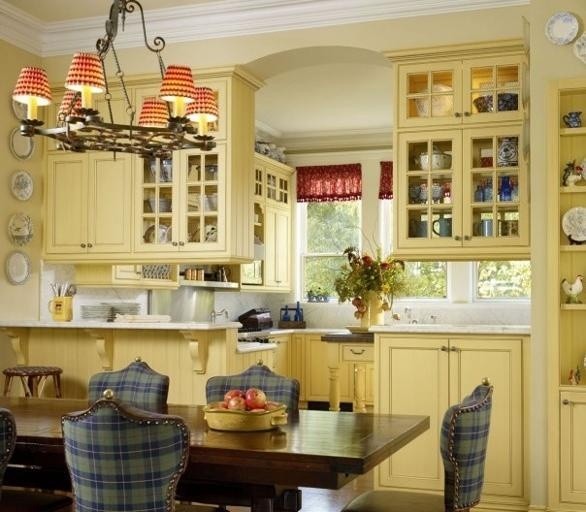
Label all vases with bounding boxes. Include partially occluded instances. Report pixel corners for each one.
[360,287,384,331]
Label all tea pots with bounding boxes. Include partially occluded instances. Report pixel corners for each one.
[216,264,231,282]
[562,112,583,127]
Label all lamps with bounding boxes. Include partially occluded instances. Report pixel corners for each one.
[11,1,217,159]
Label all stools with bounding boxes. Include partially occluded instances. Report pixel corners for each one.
[3,366,63,398]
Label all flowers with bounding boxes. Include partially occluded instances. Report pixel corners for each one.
[332,245,403,318]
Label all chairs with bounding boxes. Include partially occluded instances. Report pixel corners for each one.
[341,375,496,507]
[0,408,17,473]
[179,358,302,512]
[87,356,169,413]
[60,389,190,512]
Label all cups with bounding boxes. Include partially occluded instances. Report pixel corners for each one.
[191,268,198,281]
[499,220,517,236]
[198,267,204,281]
[185,267,191,281]
[479,219,499,236]
[433,217,452,236]
[409,218,427,237]
[256,140,288,164]
[46,296,72,322]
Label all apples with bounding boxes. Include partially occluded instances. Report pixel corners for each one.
[218,387,279,414]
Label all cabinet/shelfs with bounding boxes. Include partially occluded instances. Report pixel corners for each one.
[238,334,528,508]
[548,77,586,511]
[43,67,299,296]
[396,52,531,262]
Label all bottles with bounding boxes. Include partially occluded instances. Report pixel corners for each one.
[282,304,290,321]
[501,177,512,203]
[294,302,302,321]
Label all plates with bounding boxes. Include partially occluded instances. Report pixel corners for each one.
[572,30,586,66]
[7,127,36,161]
[5,249,31,288]
[9,98,27,121]
[144,224,168,242]
[6,212,32,247]
[166,224,216,241]
[79,301,140,322]
[114,312,172,324]
[9,170,33,202]
[543,12,579,45]
[414,83,456,117]
[561,206,586,243]
[499,141,519,167]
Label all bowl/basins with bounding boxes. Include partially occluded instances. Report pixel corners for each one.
[148,160,191,184]
[200,400,288,431]
[196,165,216,181]
[473,92,521,113]
[196,194,217,211]
[419,153,452,170]
[147,197,171,213]
